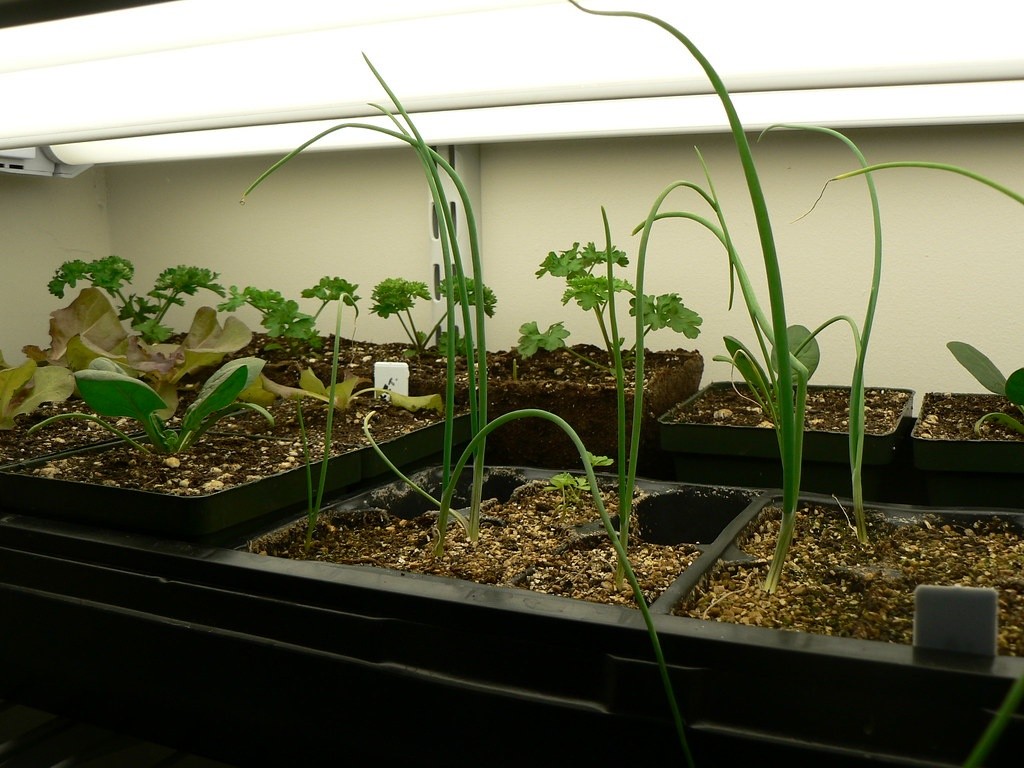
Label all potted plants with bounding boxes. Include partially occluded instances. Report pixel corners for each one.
[1,1,1024,768]
